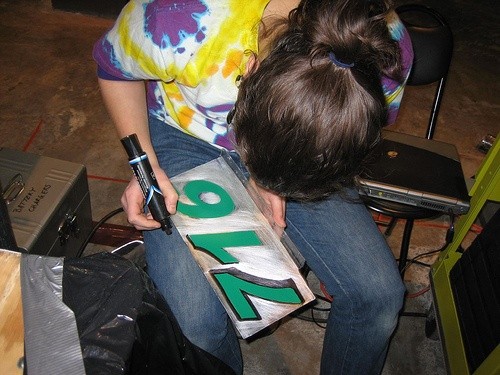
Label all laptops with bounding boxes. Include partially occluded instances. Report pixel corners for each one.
[356,127,470,215]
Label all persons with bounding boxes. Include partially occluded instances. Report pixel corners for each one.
[90,0,414,375]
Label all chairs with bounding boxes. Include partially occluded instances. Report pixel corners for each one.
[361,5,455,284]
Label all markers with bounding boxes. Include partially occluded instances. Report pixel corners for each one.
[120,132,174,236]
